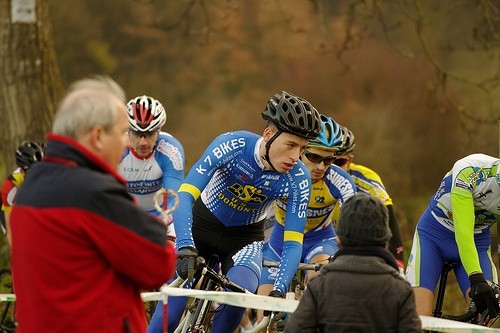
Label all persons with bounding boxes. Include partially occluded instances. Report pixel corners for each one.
[117,94,185,235]
[283,192,424,333]
[253,113,359,333]
[330,124,407,277]
[146,91,323,333]
[0,140,46,248]
[403,152,500,333]
[11,73,175,333]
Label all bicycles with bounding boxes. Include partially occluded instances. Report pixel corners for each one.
[159,254,287,333]
[261,256,335,302]
[0,267,17,333]
[427,258,500,329]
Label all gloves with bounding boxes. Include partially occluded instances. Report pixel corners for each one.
[177,246,199,283]
[468,272,500,318]
[264,290,285,324]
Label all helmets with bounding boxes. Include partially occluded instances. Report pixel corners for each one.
[332,125,355,157]
[308,113,343,150]
[15,141,46,173]
[261,90,322,140]
[126,95,166,132]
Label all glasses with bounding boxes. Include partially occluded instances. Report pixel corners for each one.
[303,150,336,165]
[131,129,159,137]
[333,156,351,167]
